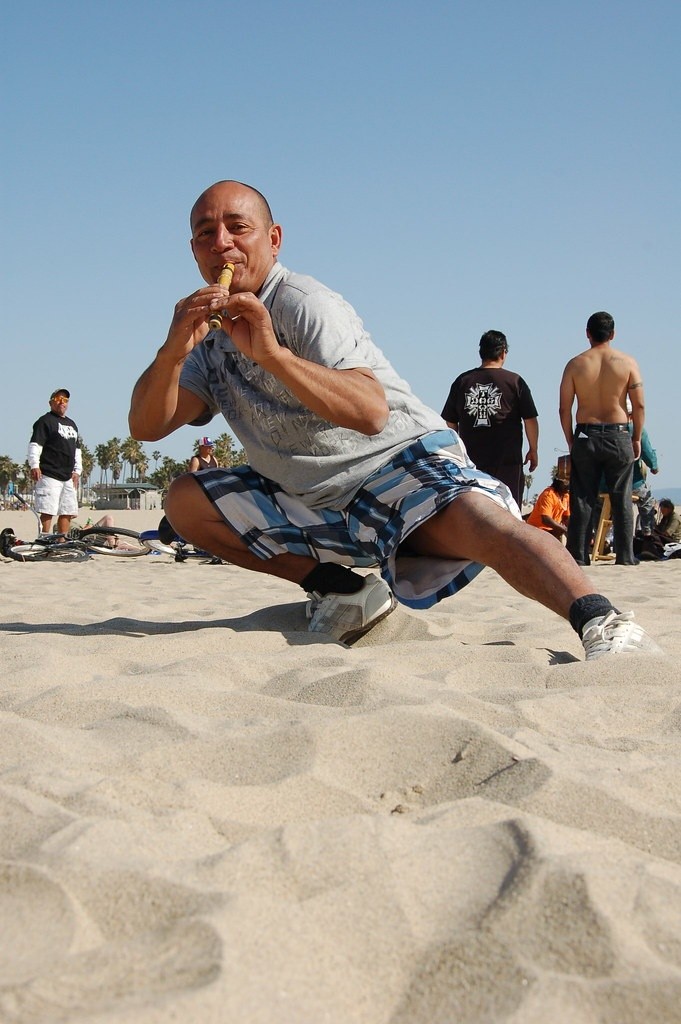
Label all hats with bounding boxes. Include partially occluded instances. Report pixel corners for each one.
[50,387,70,399]
[626,399,633,413]
[199,437,215,446]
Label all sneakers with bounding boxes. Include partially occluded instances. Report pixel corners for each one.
[305,573,398,645]
[581,609,649,661]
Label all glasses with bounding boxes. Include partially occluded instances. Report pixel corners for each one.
[51,396,69,403]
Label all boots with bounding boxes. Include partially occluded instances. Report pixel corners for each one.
[640,536,659,559]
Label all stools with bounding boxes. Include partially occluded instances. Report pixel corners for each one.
[593,494,639,562]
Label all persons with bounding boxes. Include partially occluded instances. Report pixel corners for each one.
[559,311,646,565]
[127,179,651,659]
[441,331,539,506]
[53,515,140,550]
[28,388,83,542]
[624,400,658,534]
[589,498,681,559]
[190,436,218,473]
[528,473,570,541]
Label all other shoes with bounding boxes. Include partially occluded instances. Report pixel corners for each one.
[575,559,589,566]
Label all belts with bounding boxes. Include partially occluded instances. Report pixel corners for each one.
[577,423,629,431]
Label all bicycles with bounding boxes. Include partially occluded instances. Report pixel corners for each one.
[0,492,152,562]
[138,530,224,564]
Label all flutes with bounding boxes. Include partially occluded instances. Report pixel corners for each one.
[208,262,235,331]
